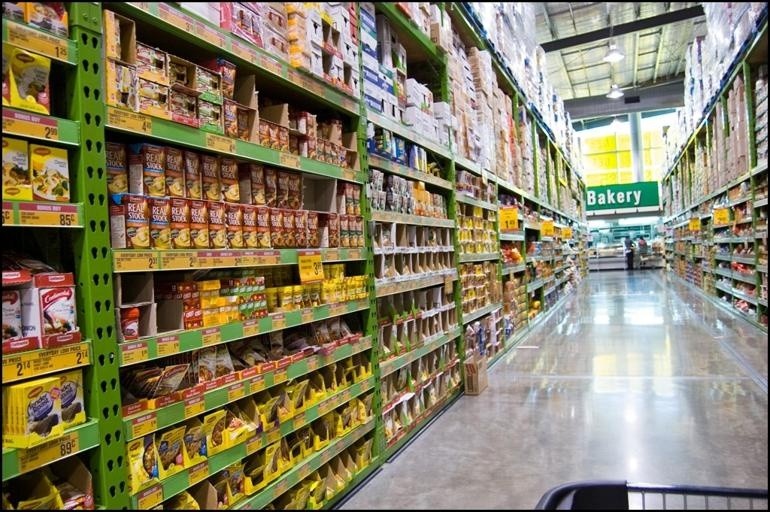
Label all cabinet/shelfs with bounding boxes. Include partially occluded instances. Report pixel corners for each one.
[656,1,768,329]
[1,1,588,511]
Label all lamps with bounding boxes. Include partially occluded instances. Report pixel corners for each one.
[602,0,625,64]
[607,65,626,100]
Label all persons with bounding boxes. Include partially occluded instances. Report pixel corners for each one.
[623,235,636,271]
[637,236,648,270]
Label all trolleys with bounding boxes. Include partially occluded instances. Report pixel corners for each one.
[537,480,769,510]
